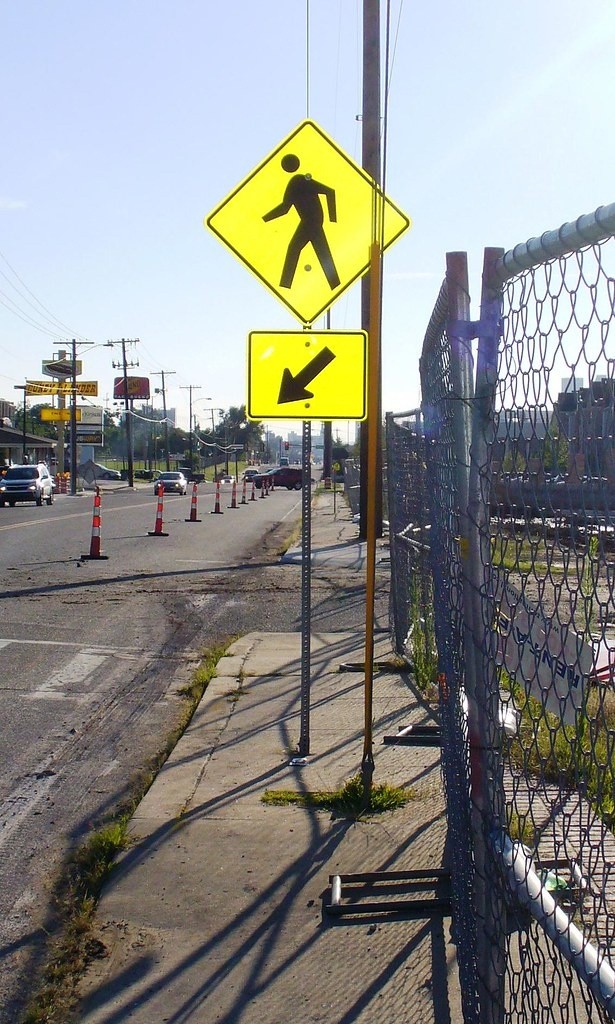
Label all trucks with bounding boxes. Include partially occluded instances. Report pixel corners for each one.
[178,467,205,483]
[280,456,290,466]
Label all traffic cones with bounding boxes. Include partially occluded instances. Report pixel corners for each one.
[134,469,162,480]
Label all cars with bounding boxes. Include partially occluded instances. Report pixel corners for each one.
[242,468,261,482]
[154,472,187,495]
[220,475,236,484]
[294,459,300,465]
[95,463,122,479]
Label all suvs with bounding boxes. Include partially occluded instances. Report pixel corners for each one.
[252,466,303,490]
[0,463,53,507]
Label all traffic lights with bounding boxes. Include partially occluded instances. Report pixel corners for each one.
[285,442,289,450]
